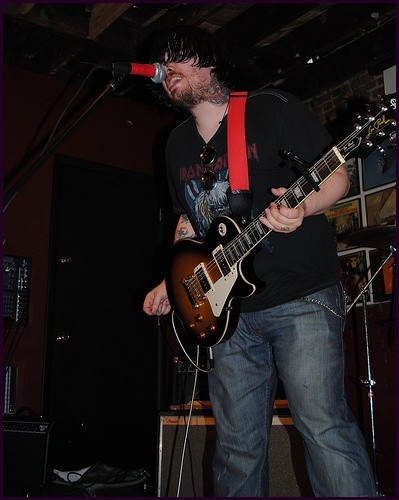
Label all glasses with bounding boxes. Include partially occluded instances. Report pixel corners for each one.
[199,145,218,190]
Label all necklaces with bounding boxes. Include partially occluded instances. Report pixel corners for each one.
[224,100,229,117]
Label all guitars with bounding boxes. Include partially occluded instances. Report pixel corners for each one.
[165,93,399,348]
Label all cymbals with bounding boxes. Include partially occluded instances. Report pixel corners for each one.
[339,225,399,249]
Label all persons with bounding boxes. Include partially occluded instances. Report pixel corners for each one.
[143,25,378,498]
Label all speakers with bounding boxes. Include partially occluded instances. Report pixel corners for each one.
[2,413,55,497]
[155,410,316,498]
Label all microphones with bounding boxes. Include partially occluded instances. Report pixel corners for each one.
[94,61,167,84]
